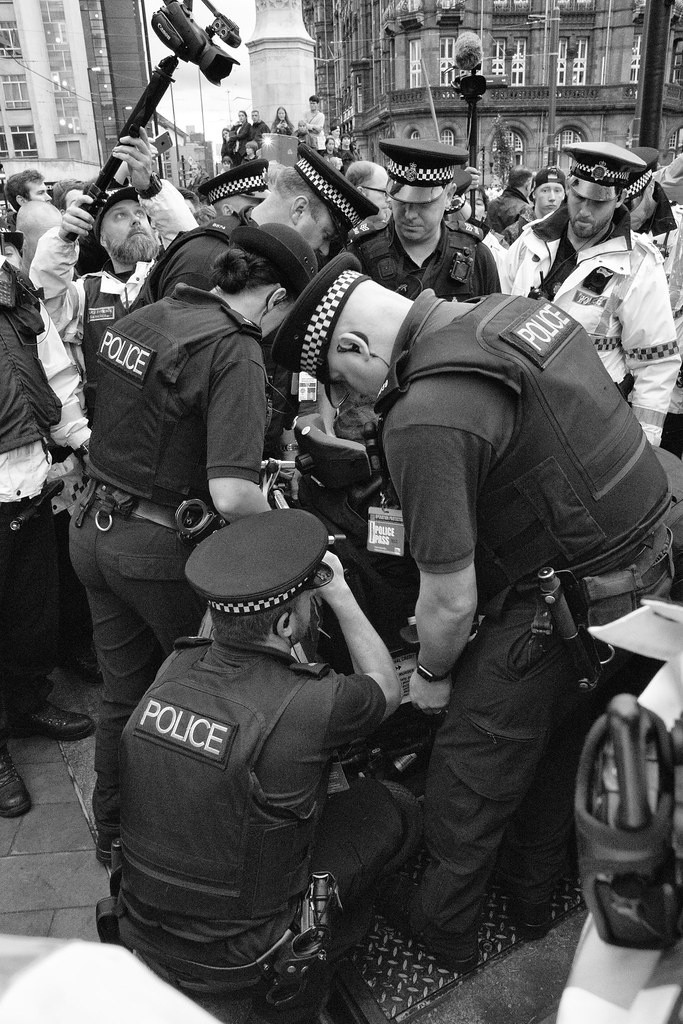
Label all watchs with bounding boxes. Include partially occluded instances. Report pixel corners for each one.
[135,172,162,198]
[416,657,452,682]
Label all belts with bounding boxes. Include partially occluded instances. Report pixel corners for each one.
[86,479,178,530]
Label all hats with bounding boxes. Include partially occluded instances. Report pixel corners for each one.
[378,139,469,203]
[620,147,658,201]
[197,159,270,203]
[443,169,471,222]
[230,224,319,286]
[529,168,566,201]
[94,185,139,240]
[270,252,370,409]
[329,126,339,135]
[293,144,380,252]
[562,142,647,202]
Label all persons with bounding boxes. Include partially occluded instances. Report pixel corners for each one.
[270,249,680,969]
[91,507,426,1024]
[1,94,682,866]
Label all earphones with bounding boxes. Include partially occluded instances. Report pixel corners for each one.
[283,618,289,629]
[347,345,360,354]
[272,295,289,307]
[616,189,623,202]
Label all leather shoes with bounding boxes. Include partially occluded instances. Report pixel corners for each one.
[0,739,29,816]
[501,857,547,939]
[378,872,481,973]
[5,700,93,741]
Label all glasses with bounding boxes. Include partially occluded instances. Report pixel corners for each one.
[358,186,388,197]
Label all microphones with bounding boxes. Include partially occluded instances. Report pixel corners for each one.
[453,31,484,71]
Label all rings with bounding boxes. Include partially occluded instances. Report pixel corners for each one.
[435,710,442,715]
